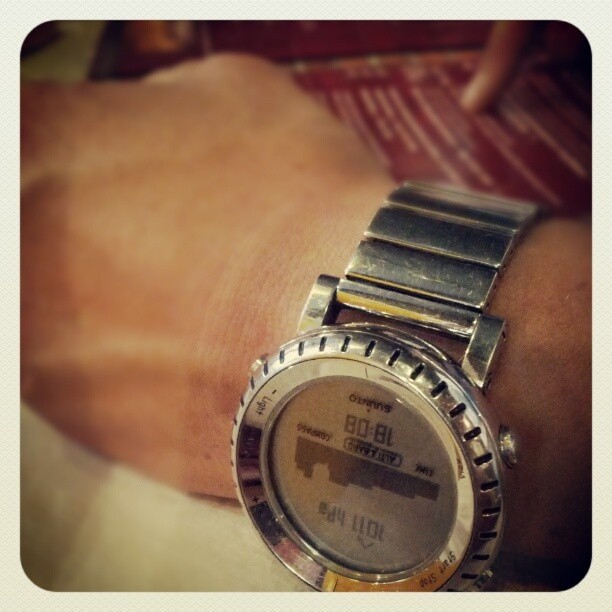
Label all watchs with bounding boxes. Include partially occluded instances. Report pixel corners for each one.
[231,174,551,592]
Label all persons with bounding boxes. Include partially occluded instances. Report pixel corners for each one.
[20,19,591,592]
